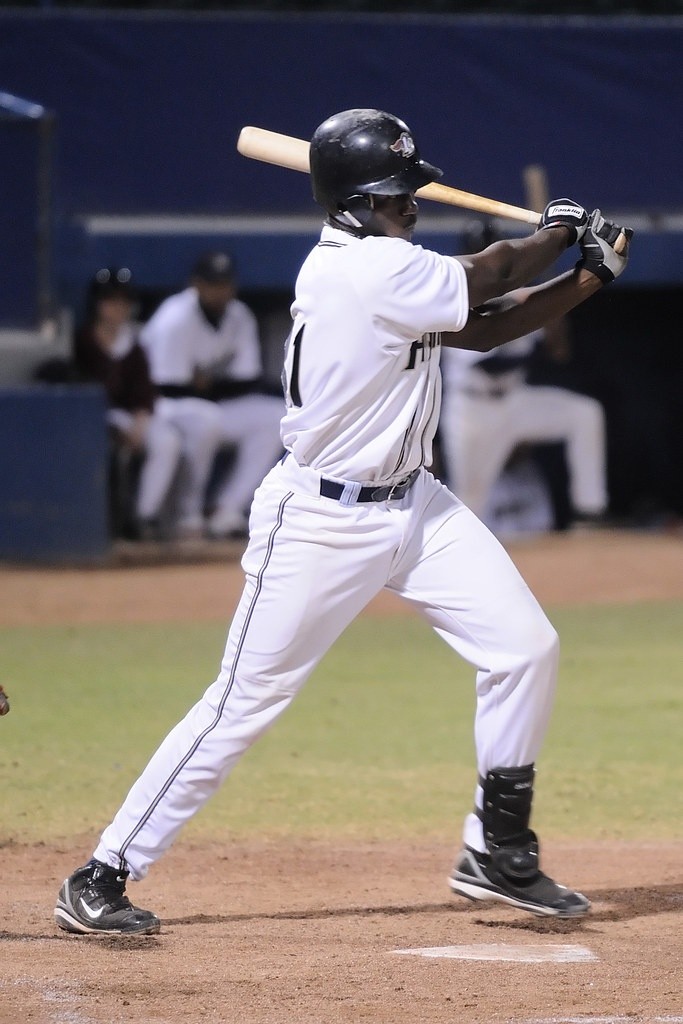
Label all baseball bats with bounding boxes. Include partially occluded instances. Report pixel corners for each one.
[236,126,627,255]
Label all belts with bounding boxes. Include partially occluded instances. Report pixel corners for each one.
[281,451,421,502]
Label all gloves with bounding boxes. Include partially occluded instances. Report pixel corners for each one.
[535,197,590,246]
[577,209,635,286]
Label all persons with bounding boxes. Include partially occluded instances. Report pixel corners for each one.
[68,263,179,543]
[53,109,636,934]
[139,245,294,536]
[440,219,613,529]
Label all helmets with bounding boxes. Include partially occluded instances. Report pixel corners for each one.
[197,252,238,281]
[309,109,445,213]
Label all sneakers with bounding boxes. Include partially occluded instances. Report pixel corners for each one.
[449,844,592,916]
[53,864,161,933]
